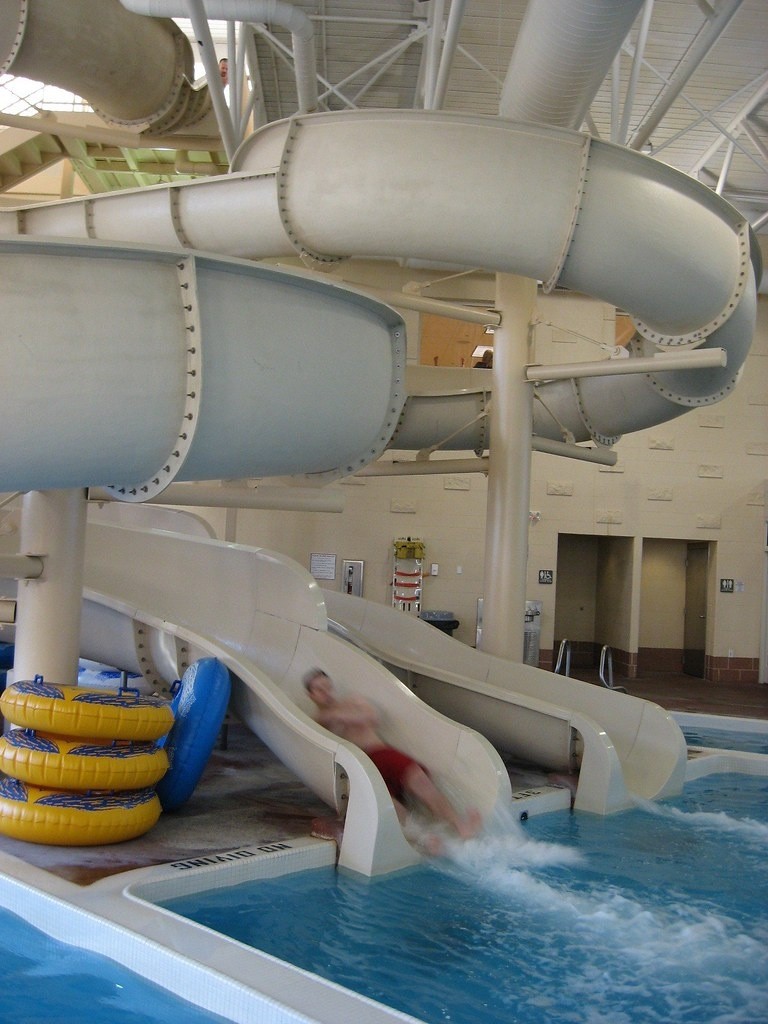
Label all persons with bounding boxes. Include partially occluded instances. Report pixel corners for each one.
[473,350,493,368]
[219,57,251,92]
[303,668,483,860]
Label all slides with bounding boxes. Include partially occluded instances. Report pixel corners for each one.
[0,1,757,880]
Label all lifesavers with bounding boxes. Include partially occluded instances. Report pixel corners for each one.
[0,774,162,845]
[0,727,167,790]
[165,656,232,811]
[1,676,172,739]
